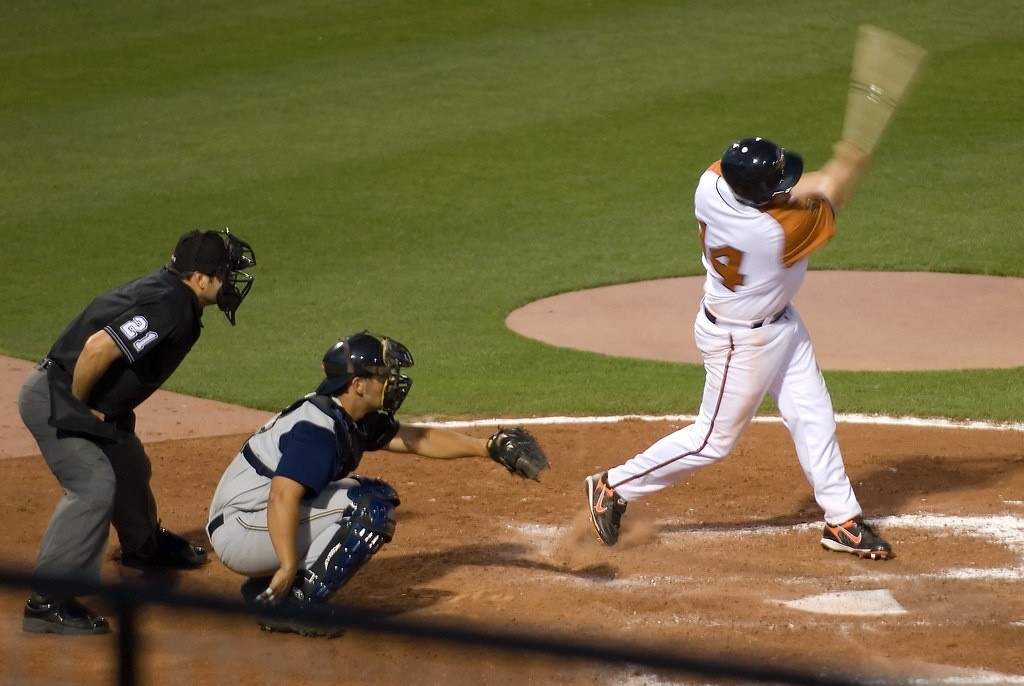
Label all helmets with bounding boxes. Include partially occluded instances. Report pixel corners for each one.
[720,139,804,198]
[315,334,394,394]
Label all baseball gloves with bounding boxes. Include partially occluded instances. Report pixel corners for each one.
[487,428,547,481]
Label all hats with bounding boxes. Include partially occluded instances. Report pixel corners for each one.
[170,229,228,274]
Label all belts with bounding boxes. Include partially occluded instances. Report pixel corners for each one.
[704,302,789,328]
[208,512,224,539]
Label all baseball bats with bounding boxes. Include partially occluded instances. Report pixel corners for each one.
[839,22,927,154]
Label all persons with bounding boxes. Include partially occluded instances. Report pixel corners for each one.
[205,331,521,641]
[585,139,894,560]
[14,226,257,633]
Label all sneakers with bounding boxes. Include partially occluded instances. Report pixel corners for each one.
[259,599,340,637]
[821,516,892,561]
[583,470,627,546]
[24,594,111,637]
[119,526,208,570]
